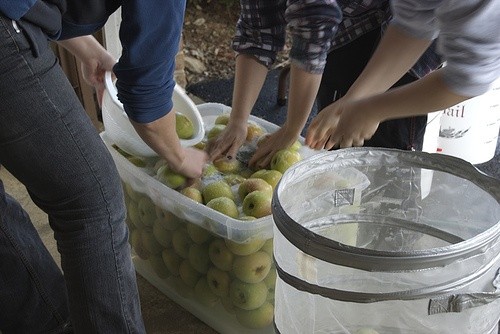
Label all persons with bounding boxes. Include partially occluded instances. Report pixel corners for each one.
[216,0,426,165]
[0,0,212,333]
[305,0,500,150]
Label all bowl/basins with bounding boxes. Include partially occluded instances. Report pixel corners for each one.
[101,69,205,159]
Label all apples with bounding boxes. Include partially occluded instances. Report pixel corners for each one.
[110,112,301,330]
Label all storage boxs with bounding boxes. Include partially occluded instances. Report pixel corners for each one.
[97,101,372,333]
